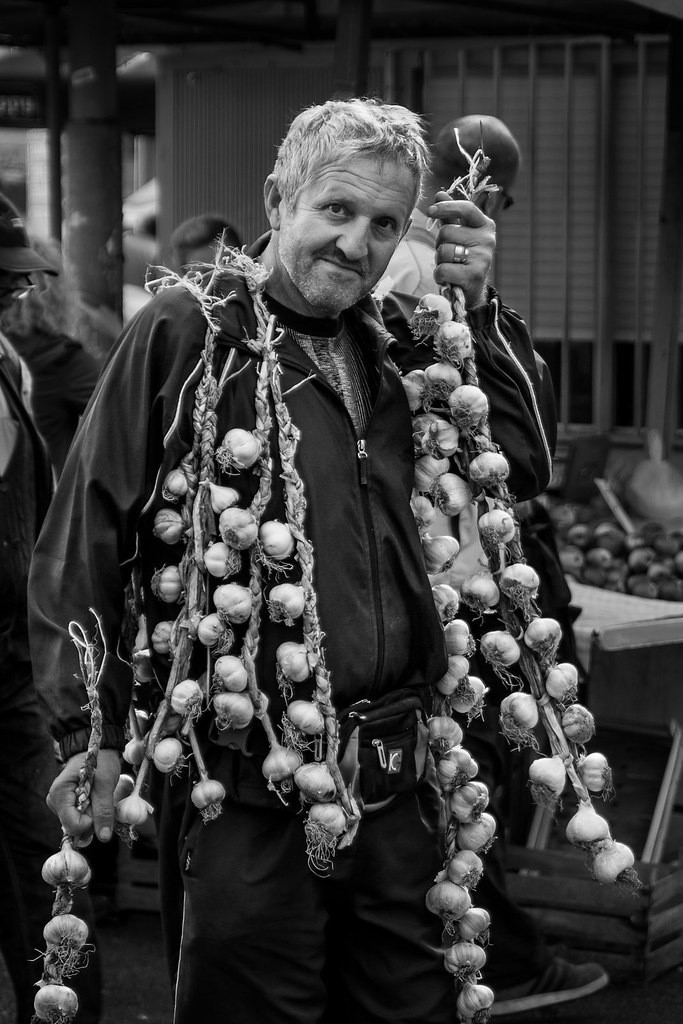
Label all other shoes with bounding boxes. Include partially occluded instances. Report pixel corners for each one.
[486,956,609,1017]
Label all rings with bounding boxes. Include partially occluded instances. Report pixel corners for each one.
[454,244,468,263]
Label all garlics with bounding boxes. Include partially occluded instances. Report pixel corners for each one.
[396,293,641,1024]
[34,426,347,1024]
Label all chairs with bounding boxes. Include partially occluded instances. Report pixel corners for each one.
[544,436,608,503]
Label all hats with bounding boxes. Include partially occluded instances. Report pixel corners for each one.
[0,192,59,278]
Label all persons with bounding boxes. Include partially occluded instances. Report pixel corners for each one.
[0,196,105,1024]
[27,98,609,1024]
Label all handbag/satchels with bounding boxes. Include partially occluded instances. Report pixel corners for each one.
[332,687,432,812]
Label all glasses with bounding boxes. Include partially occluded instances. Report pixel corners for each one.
[0,276,37,300]
[499,190,514,210]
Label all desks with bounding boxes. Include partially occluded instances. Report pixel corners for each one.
[510,575,683,879]
